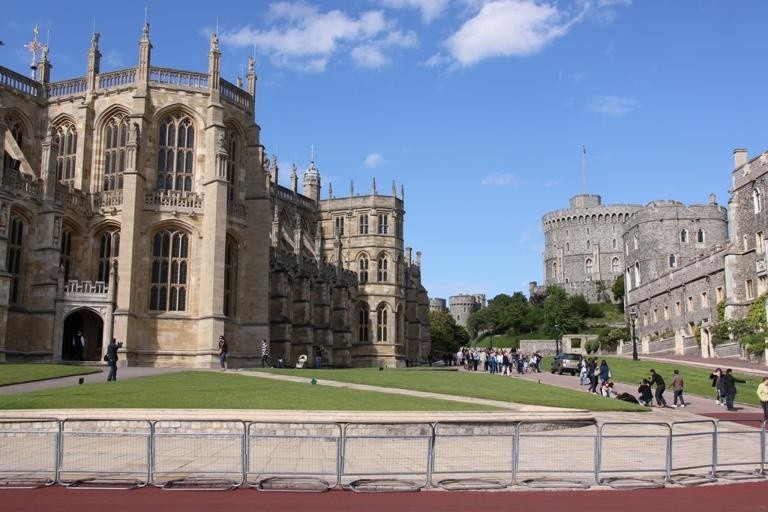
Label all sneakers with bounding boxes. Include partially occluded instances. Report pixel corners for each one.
[670,405,676,408]
[680,405,684,408]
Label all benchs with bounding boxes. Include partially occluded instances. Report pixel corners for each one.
[284,360,291,368]
[316,362,345,369]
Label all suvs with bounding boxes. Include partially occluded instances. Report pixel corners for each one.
[550,353,583,376]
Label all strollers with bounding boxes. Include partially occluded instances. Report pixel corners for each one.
[295,354,307,369]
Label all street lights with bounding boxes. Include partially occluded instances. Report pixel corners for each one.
[554,323,559,355]
[489,328,493,348]
[629,309,638,361]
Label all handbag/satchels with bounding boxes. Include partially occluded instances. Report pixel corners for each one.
[104,355,108,361]
[593,368,600,376]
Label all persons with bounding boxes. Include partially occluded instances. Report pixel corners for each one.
[576,355,639,405]
[708,368,726,407]
[216,335,227,372]
[442,352,447,366]
[260,338,274,371]
[297,355,305,364]
[715,373,728,407]
[452,345,544,377]
[667,369,684,408]
[105,337,123,383]
[276,356,284,369]
[427,351,432,367]
[637,378,653,406]
[315,346,322,369]
[647,368,667,408]
[722,368,748,411]
[71,329,85,360]
[755,376,768,425]
[447,351,452,367]
